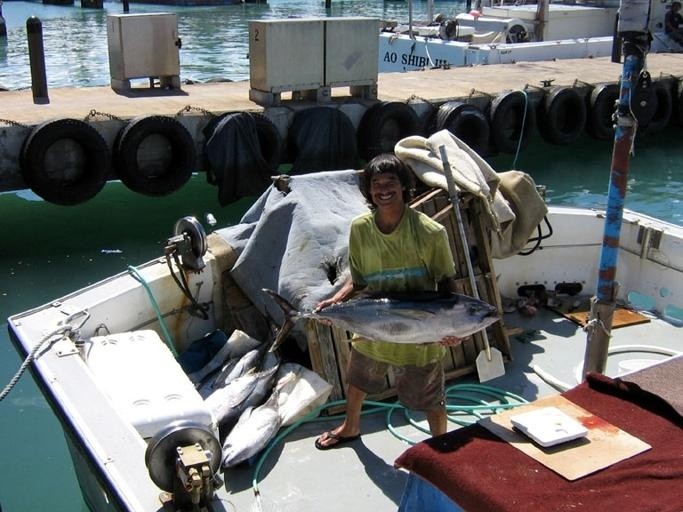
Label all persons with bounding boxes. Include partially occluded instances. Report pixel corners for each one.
[306,151,472,453]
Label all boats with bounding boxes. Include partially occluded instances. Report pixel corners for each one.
[7,207,683,512]
[378,0,683,74]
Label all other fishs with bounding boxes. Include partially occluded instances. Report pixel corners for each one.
[260,288,503,353]
[188,305,303,469]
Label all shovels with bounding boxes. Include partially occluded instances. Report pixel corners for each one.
[439,145,506,384]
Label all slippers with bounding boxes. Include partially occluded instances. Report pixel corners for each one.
[315,430,361,450]
